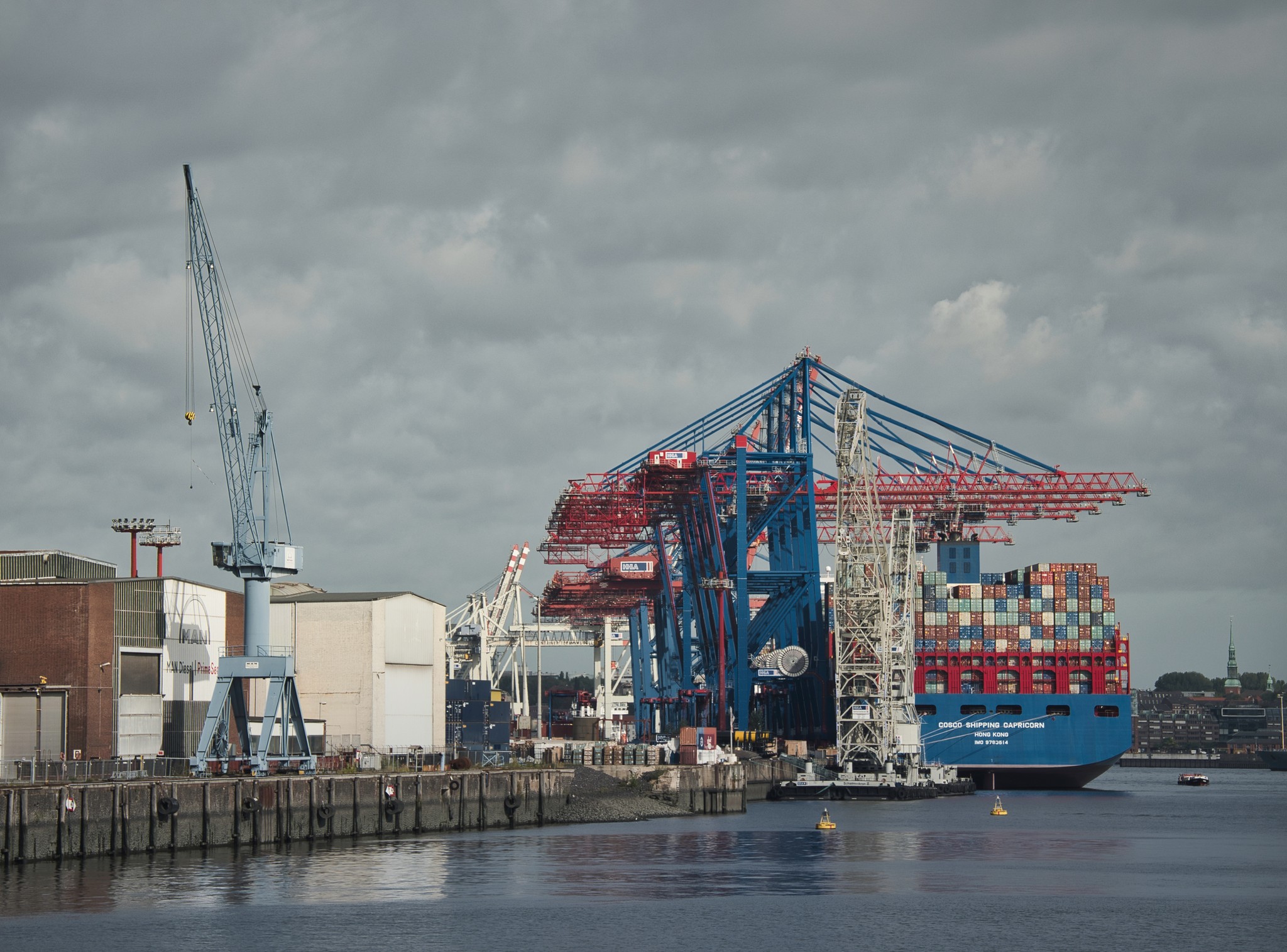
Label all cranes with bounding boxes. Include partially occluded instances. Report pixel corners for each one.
[444,348,1155,802]
[171,164,316,778]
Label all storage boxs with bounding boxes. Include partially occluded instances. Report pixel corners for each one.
[14,679,716,786]
[820,563,1116,694]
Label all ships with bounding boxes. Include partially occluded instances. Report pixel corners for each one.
[823,559,1133,791]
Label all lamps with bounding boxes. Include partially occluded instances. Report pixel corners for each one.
[99,662,110,668]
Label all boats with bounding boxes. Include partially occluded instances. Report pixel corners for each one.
[1178,774,1210,787]
